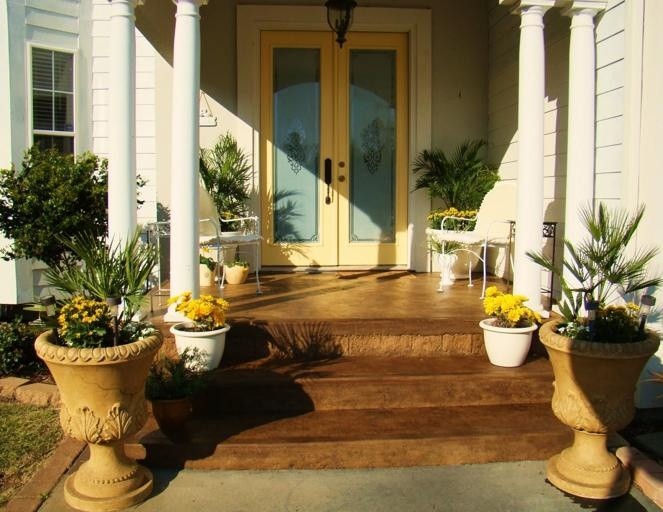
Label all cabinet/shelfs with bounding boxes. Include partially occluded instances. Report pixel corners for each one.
[504,220,558,309]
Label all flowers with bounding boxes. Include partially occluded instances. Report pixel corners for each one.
[24,223,159,349]
[521,198,663,343]
[158,290,230,331]
[481,286,542,327]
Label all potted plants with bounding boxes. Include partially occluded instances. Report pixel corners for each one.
[145,347,209,432]
[406,137,500,285]
[198,130,251,286]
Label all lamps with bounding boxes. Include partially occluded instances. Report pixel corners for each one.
[324,0,359,49]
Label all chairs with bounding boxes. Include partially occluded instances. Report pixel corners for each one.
[436,181,518,300]
[199,173,264,300]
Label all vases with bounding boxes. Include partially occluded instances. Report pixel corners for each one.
[479,318,538,368]
[539,316,660,499]
[169,322,230,373]
[35,319,164,512]
